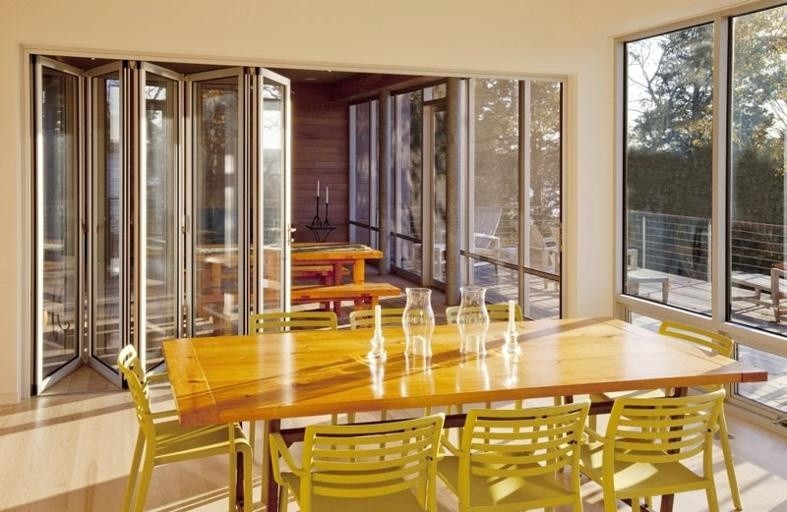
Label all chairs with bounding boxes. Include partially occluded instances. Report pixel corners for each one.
[732,264,787,323]
[412,204,560,305]
[625,248,671,302]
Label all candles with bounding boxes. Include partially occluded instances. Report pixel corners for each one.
[314,176,330,205]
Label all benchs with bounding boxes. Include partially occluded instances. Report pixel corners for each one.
[194,240,402,335]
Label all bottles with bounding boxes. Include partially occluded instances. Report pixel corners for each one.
[402,287,435,360]
[368,305,387,363]
[503,300,521,355]
[456,285,491,357]
